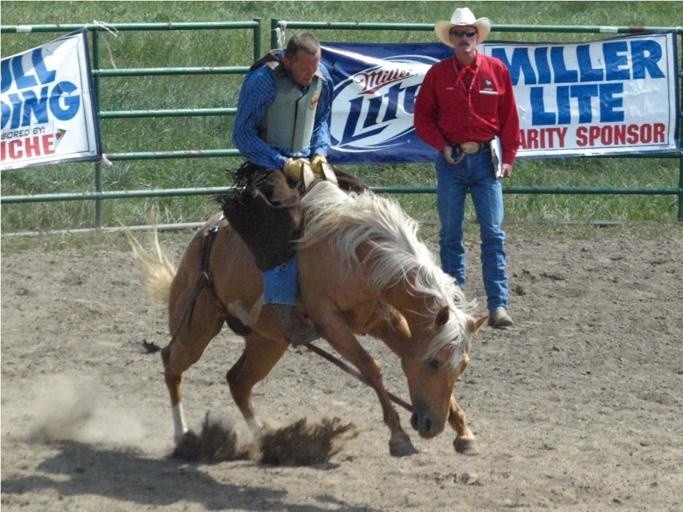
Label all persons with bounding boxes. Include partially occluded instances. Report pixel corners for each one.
[411,6,520,330]
[229,30,372,344]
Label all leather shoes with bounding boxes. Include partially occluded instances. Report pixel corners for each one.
[489,306,512,325]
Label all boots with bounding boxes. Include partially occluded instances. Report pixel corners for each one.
[273,305,319,344]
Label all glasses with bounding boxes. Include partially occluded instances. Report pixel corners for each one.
[452,30,475,37]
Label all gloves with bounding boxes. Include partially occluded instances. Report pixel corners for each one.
[283,157,311,181]
[311,156,327,173]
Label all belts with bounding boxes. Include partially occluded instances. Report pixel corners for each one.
[453,142,489,153]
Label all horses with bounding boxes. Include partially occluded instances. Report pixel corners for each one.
[111,177,492,457]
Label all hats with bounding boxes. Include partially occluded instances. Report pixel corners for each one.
[435,7,491,47]
